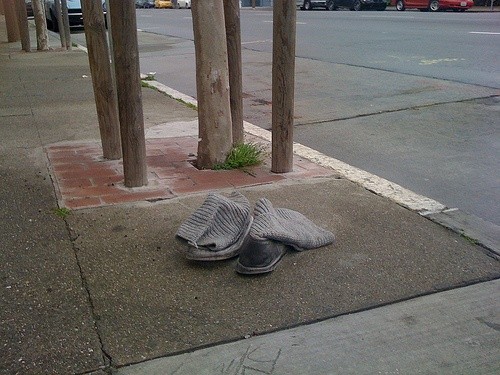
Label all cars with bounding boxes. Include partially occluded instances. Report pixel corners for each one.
[46,0,110,33]
[390,0,475,12]
[296,0,327,10]
[136,0,191,9]
[325,0,387,12]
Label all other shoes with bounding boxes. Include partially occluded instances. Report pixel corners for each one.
[174,189,336,276]
